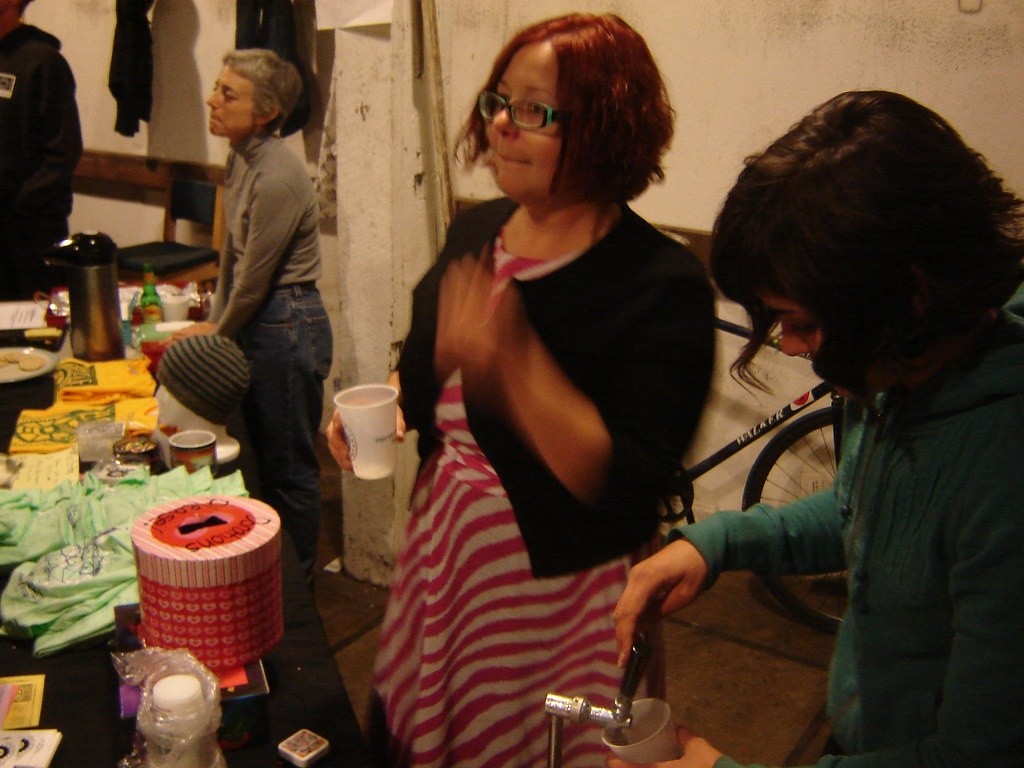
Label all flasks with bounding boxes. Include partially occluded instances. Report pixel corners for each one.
[65,231,126,363]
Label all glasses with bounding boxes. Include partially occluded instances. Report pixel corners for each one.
[478,92,564,129]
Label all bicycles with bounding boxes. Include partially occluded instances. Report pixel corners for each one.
[651,307,850,635]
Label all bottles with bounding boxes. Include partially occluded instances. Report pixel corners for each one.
[141,263,163,323]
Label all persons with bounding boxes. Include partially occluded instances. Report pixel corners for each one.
[155,336,247,465]
[325,14,715,768]
[0,0,84,302]
[159,48,334,628]
[605,90,1024,768]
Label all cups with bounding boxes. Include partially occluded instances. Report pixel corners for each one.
[334,385,399,479]
[150,675,220,768]
[601,697,678,764]
[114,434,160,476]
[169,432,217,475]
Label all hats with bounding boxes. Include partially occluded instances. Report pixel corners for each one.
[155,333,249,426]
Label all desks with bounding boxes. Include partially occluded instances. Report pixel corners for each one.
[0,320,377,768]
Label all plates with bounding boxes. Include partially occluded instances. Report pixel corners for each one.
[0,347,58,382]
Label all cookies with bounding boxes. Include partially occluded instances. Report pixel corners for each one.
[0,351,43,370]
